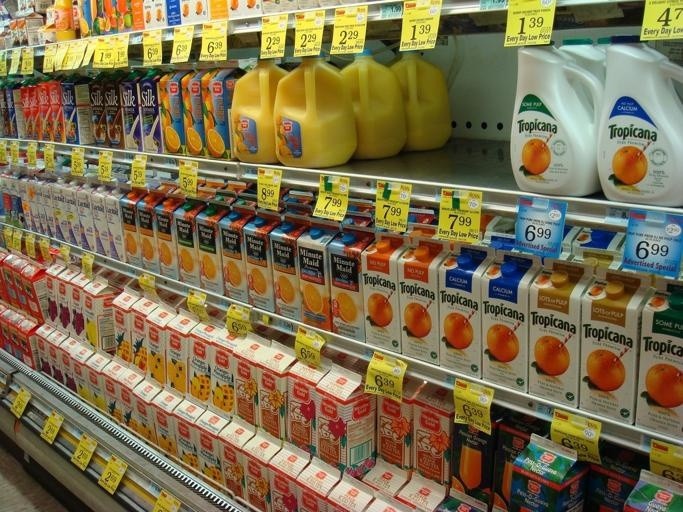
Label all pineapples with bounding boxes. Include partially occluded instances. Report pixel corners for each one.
[180,444,198,468]
[159,433,175,451]
[190,371,211,402]
[138,422,150,437]
[123,410,138,432]
[149,350,165,383]
[168,358,186,395]
[132,337,147,373]
[107,400,123,422]
[212,381,234,412]
[201,456,221,482]
[115,330,131,361]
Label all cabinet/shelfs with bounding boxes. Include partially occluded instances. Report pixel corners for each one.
[0,1,682,512]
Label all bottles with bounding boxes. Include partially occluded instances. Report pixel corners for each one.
[295,455,342,512]
[437,241,496,381]
[210,326,247,416]
[70,269,96,338]
[219,415,256,498]
[71,341,96,401]
[195,207,227,295]
[146,302,176,384]
[0,301,39,372]
[287,352,333,457]
[119,69,143,150]
[438,488,487,512]
[139,68,163,155]
[269,219,310,321]
[136,193,166,275]
[232,330,271,428]
[34,319,57,377]
[0,249,47,324]
[188,316,223,403]
[200,65,248,160]
[281,189,373,228]
[46,325,70,388]
[394,471,447,512]
[327,471,375,511]
[154,197,185,282]
[242,428,282,512]
[623,468,683,512]
[174,201,206,287]
[481,248,541,395]
[255,339,297,442]
[0,72,95,145]
[181,66,213,158]
[0,168,127,263]
[269,440,310,512]
[154,171,258,209]
[44,258,67,326]
[118,363,146,430]
[493,416,551,511]
[527,261,596,412]
[296,220,342,333]
[398,237,452,369]
[362,457,409,496]
[103,354,129,421]
[83,275,122,352]
[243,209,282,313]
[364,491,413,512]
[131,374,163,447]
[159,69,188,156]
[578,265,652,428]
[59,264,81,333]
[361,230,411,354]
[172,392,208,469]
[59,331,83,394]
[194,403,231,491]
[406,206,625,275]
[450,411,500,507]
[87,70,107,148]
[84,347,112,413]
[510,432,590,512]
[634,271,683,440]
[128,293,160,375]
[150,384,184,457]
[218,208,255,305]
[121,189,143,269]
[410,382,455,483]
[376,376,425,468]
[165,307,200,393]
[327,228,376,342]
[112,285,142,363]
[314,362,373,475]
[103,68,124,150]
[77,1,261,37]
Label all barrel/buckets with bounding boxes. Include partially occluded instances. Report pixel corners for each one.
[341,50,406,161]
[597,35,683,206]
[231,56,290,165]
[388,50,451,151]
[273,54,358,168]
[510,40,605,197]
[558,36,605,83]
